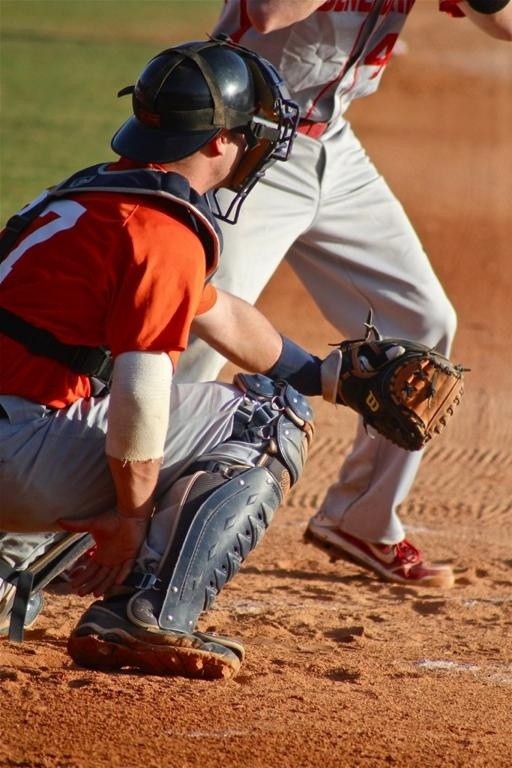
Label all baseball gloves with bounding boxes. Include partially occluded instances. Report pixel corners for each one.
[320,333,464,451]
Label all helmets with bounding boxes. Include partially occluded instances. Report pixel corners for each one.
[112,31,301,225]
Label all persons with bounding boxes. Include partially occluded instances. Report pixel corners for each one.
[0,31,465,681]
[174,0,512,590]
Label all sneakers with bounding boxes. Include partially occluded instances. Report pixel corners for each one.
[68,600,245,680]
[304,511,455,589]
[0,591,43,638]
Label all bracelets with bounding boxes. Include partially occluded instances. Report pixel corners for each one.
[268,334,322,397]
